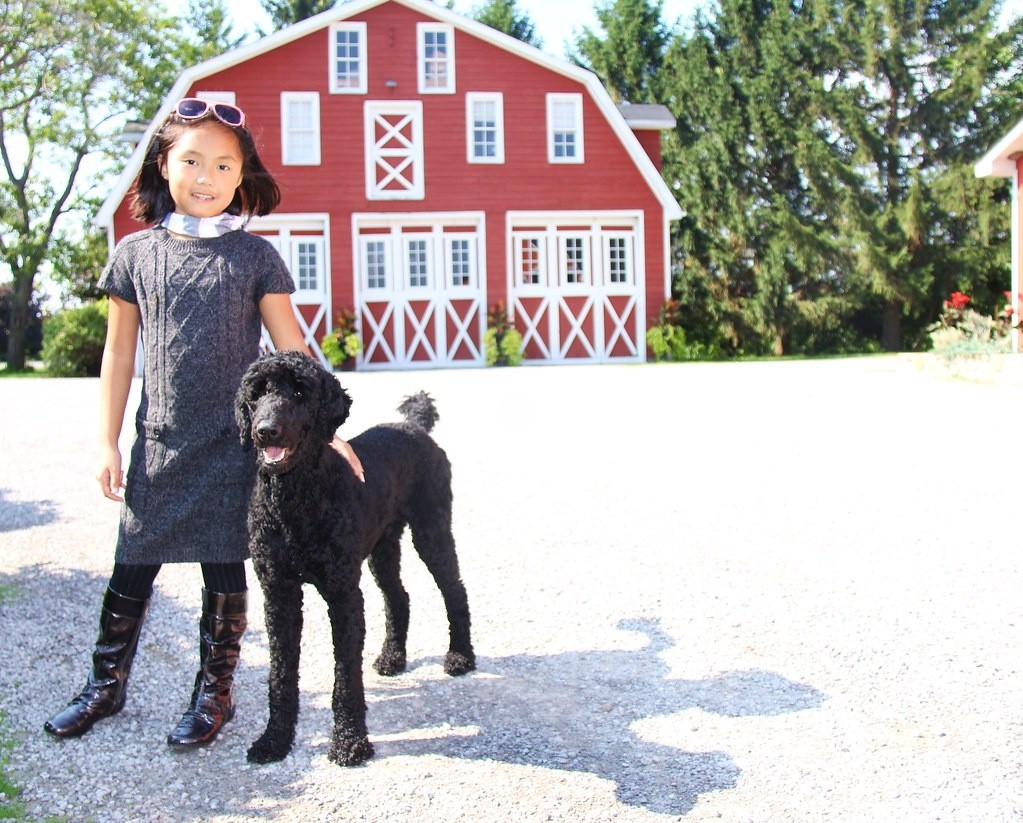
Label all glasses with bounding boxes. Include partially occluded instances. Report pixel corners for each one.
[157,98,246,141]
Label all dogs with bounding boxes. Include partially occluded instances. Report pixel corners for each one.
[233,351,476,768]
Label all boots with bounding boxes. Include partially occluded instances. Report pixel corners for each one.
[167,586,249,748]
[44,586,154,738]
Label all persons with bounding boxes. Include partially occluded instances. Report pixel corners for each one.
[44,110,365,750]
